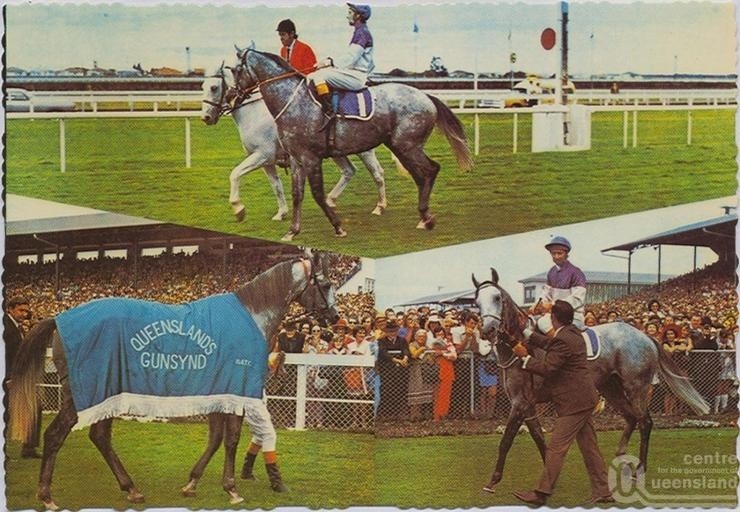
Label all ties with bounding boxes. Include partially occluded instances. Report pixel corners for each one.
[286,47,291,63]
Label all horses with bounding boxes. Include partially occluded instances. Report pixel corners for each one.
[199,60,413,222]
[471,267,712,494]
[222,39,473,242]
[7,250,342,511]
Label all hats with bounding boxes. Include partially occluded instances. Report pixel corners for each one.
[283,321,298,329]
[424,314,444,331]
[274,18,297,32]
[383,320,400,334]
[375,311,386,320]
[544,235,572,252]
[332,319,351,334]
[346,2,373,21]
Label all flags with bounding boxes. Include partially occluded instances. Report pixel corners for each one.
[414,24,419,31]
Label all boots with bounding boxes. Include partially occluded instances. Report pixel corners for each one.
[239,453,260,482]
[315,91,341,137]
[265,462,295,497]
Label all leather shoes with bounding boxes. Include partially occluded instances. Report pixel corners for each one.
[578,493,617,506]
[508,487,548,508]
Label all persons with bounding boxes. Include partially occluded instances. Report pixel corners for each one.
[274,19,318,75]
[611,82,618,93]
[535,82,542,93]
[527,80,535,96]
[4,238,739,505]
[312,3,374,132]
